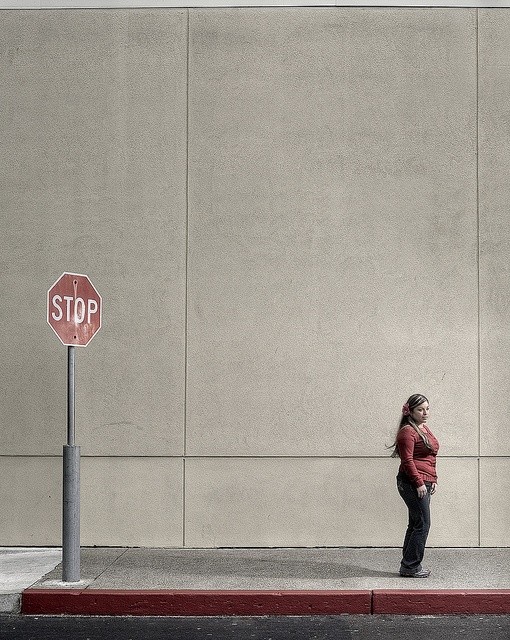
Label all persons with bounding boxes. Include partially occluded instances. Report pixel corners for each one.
[384,393,440,578]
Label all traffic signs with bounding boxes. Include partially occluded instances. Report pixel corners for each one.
[46,272,102,347]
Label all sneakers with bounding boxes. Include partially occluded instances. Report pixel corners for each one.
[400,568,431,578]
